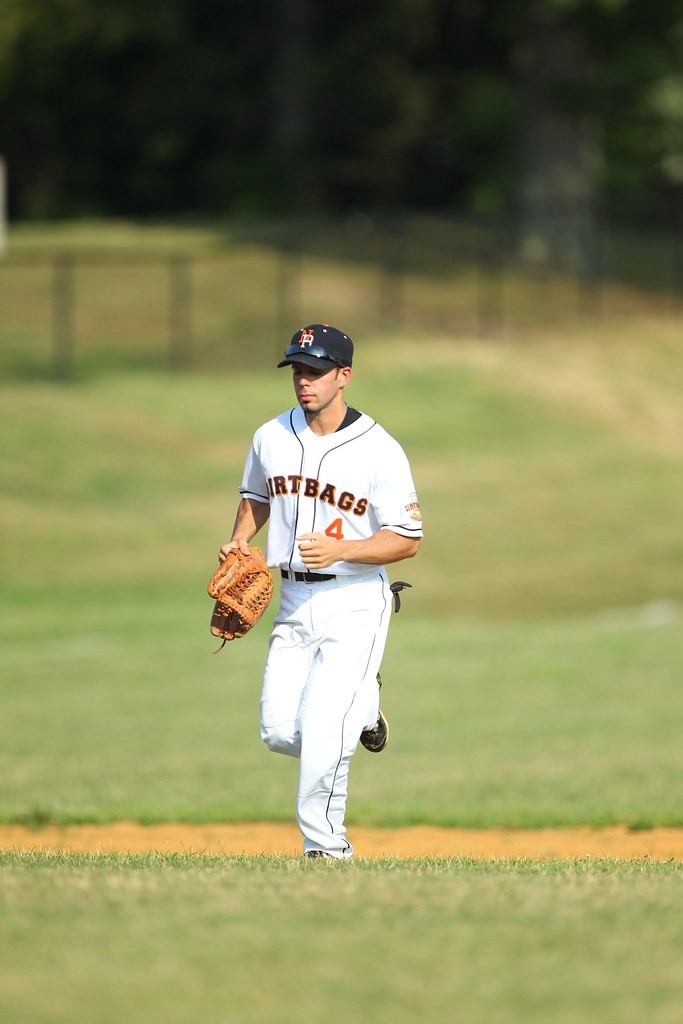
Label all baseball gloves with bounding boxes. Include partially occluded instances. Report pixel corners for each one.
[208,547,275,641]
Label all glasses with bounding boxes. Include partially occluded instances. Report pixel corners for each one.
[285,345,346,369]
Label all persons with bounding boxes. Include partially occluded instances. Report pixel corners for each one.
[217,324,423,859]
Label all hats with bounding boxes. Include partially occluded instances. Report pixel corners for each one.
[276,322,354,371]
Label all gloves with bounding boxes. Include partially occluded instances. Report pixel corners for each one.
[390,581,412,612]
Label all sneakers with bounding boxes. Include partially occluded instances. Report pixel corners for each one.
[359,673,389,753]
[307,850,330,858]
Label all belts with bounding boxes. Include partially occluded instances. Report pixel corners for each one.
[281,568,336,584]
